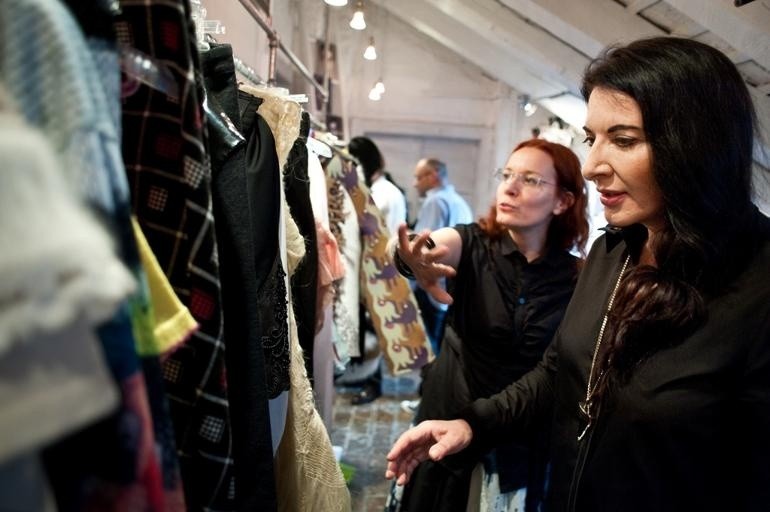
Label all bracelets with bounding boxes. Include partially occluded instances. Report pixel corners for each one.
[394,233,436,281]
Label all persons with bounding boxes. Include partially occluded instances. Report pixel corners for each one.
[413,159,476,348]
[349,134,406,259]
[392,140,596,512]
[386,33,770,512]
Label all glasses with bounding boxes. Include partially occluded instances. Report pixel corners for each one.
[490,166,566,189]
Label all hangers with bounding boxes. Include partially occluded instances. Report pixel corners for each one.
[104,0,333,158]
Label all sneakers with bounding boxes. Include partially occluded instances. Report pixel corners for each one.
[351,383,420,422]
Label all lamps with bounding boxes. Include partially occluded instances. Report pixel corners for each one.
[363,36,376,60]
[369,77,384,101]
[349,0,366,30]
